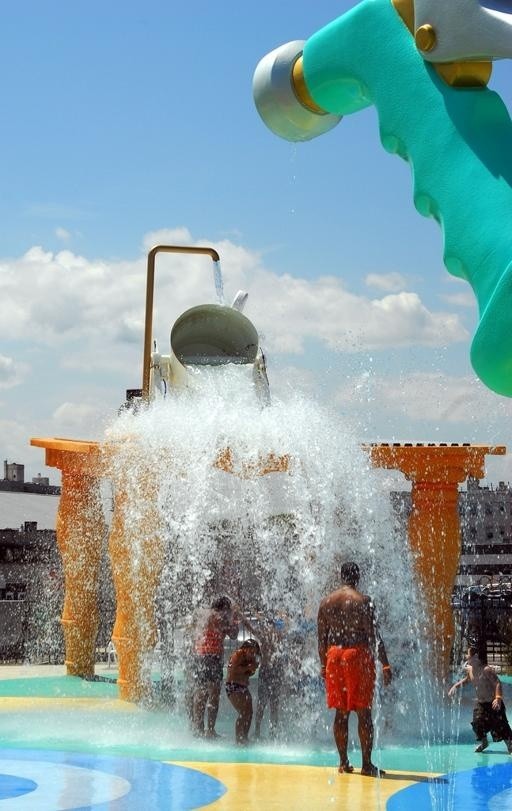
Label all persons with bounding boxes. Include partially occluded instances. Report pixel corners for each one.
[448,646,512,754]
[316,561,393,776]
[180,578,317,748]
[378,638,420,733]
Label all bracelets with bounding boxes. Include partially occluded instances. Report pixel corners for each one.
[494,695,503,700]
[321,665,326,670]
[382,664,391,671]
